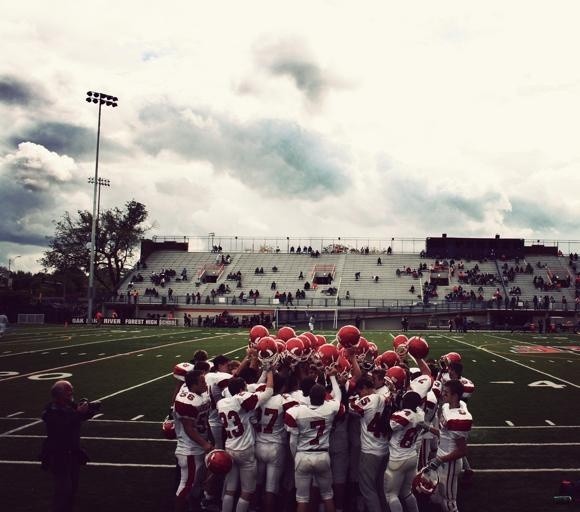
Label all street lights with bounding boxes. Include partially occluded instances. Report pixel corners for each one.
[82,87,122,325]
[9,255,22,270]
[86,174,110,265]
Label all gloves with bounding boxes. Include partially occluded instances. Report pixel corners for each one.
[415,421,431,432]
[427,456,441,471]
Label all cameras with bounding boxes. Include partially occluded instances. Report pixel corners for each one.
[78,398,102,415]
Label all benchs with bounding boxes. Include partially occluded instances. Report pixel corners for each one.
[107,252,579,308]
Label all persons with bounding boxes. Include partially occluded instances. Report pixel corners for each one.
[38,379,101,512]
[0,312,8,339]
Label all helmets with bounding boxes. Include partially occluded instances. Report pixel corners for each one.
[203,447,233,474]
[161,414,176,440]
[247,323,462,389]
[410,474,440,498]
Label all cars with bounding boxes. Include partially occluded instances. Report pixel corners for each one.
[527,315,575,333]
